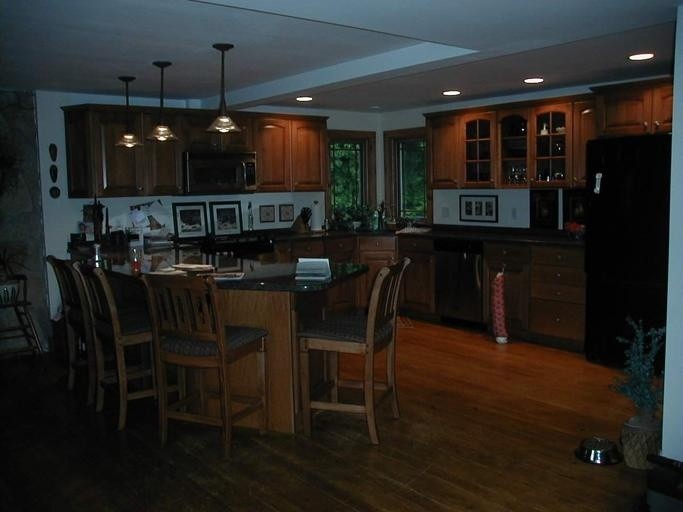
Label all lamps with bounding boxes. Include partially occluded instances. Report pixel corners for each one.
[113,75,144,147]
[204,43,244,134]
[146,62,179,143]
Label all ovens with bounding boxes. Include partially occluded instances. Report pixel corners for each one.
[433,238,482,324]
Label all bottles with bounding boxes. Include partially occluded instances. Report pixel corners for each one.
[92,243,102,263]
[248,201,254,232]
[129,241,143,272]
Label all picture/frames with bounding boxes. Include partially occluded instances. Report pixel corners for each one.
[259,205,275,223]
[209,200,243,237]
[172,202,209,240]
[279,204,294,222]
[459,194,499,223]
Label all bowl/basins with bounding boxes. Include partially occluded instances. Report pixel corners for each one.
[575,435,625,466]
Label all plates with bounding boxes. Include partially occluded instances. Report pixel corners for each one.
[148,268,188,279]
[172,262,213,272]
[195,272,246,282]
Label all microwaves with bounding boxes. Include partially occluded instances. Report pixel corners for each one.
[182,150,257,194]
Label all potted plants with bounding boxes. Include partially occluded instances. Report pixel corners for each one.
[338,204,363,234]
[606,317,665,470]
[362,203,379,231]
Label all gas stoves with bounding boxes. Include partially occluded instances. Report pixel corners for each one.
[175,233,266,247]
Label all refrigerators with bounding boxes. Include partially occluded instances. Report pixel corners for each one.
[584,133,672,378]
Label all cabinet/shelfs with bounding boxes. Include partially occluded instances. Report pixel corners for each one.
[179,107,255,154]
[422,93,598,191]
[253,111,329,191]
[61,105,180,199]
[589,75,673,135]
[1,274,47,376]
